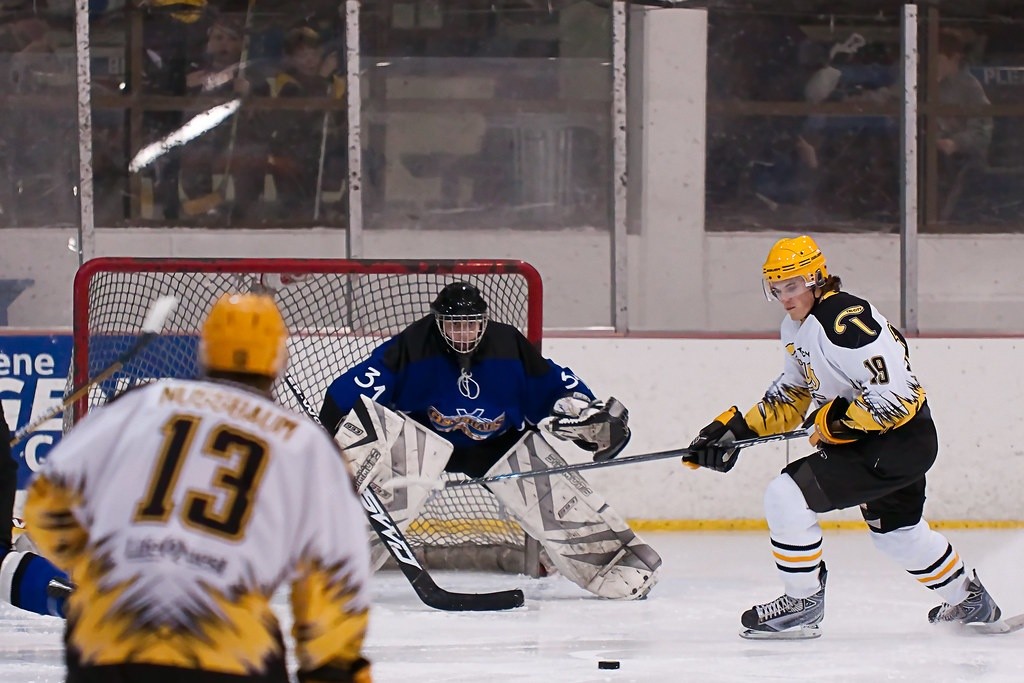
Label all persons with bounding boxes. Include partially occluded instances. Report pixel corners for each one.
[144,1,348,218]
[683,235,1002,641]
[852,25,994,227]
[319,281,663,611]
[22,294,371,682]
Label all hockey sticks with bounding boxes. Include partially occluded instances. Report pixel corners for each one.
[281,369,527,611]
[7,294,181,446]
[381,425,808,494]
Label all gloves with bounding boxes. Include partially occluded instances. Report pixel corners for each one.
[297,657,371,683]
[682,406,759,473]
[801,395,858,451]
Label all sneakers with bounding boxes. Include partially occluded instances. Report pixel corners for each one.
[739,560,827,640]
[928,568,1010,634]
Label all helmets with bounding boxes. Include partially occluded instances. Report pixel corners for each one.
[200,294,288,378]
[762,235,828,302]
[430,282,488,357]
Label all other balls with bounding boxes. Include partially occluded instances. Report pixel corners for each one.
[597,660,621,671]
